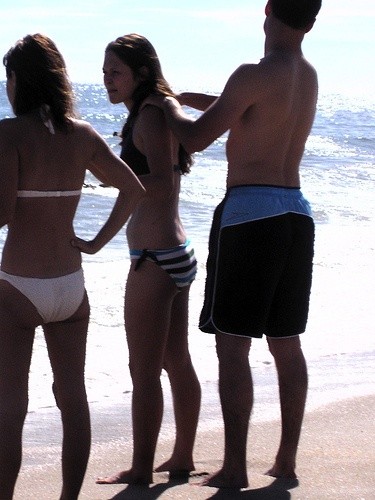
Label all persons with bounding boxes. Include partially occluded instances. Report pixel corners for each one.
[95,34,201,484]
[141,0,323,489]
[0,33,146,500]
